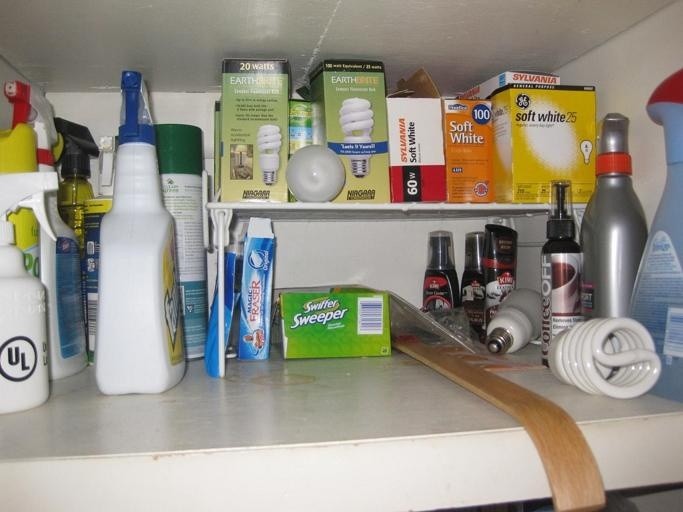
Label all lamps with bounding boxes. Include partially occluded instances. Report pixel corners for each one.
[581,141,592,164]
[486,286,547,355]
[545,317,662,401]
[285,144,346,202]
[339,98,374,179]
[257,125,281,185]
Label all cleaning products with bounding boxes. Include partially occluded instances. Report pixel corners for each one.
[3,81,88,380]
[53,114,101,339]
[93,71,187,395]
[626,68,683,403]
[0,171,60,415]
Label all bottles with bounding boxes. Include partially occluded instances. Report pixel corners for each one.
[420,230,460,312]
[460,232,487,332]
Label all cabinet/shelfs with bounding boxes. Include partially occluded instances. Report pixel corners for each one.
[2,3,683,508]
